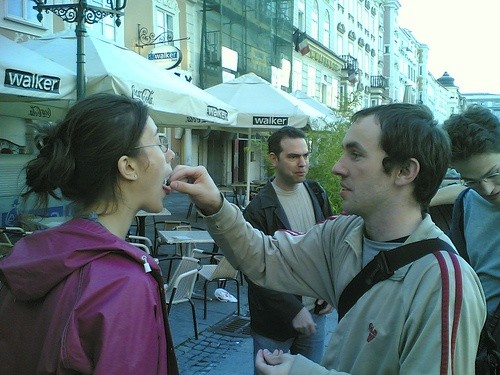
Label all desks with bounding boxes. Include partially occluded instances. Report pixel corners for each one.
[158,231,214,257]
[231,184,259,204]
[135,208,171,252]
[31,216,72,229]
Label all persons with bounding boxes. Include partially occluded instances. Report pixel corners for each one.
[440,104,500,374]
[243,128,336,375]
[168,101,488,375]
[0,94,182,375]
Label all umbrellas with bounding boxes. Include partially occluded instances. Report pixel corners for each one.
[0,32,87,103]
[289,87,351,133]
[18,24,239,129]
[202,71,321,206]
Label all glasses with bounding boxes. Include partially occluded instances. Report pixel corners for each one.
[129,134,169,153]
[460,170,500,187]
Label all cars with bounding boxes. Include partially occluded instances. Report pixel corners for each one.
[428,168,467,232]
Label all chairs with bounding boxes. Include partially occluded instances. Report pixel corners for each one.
[0,226,32,258]
[124,180,268,341]
